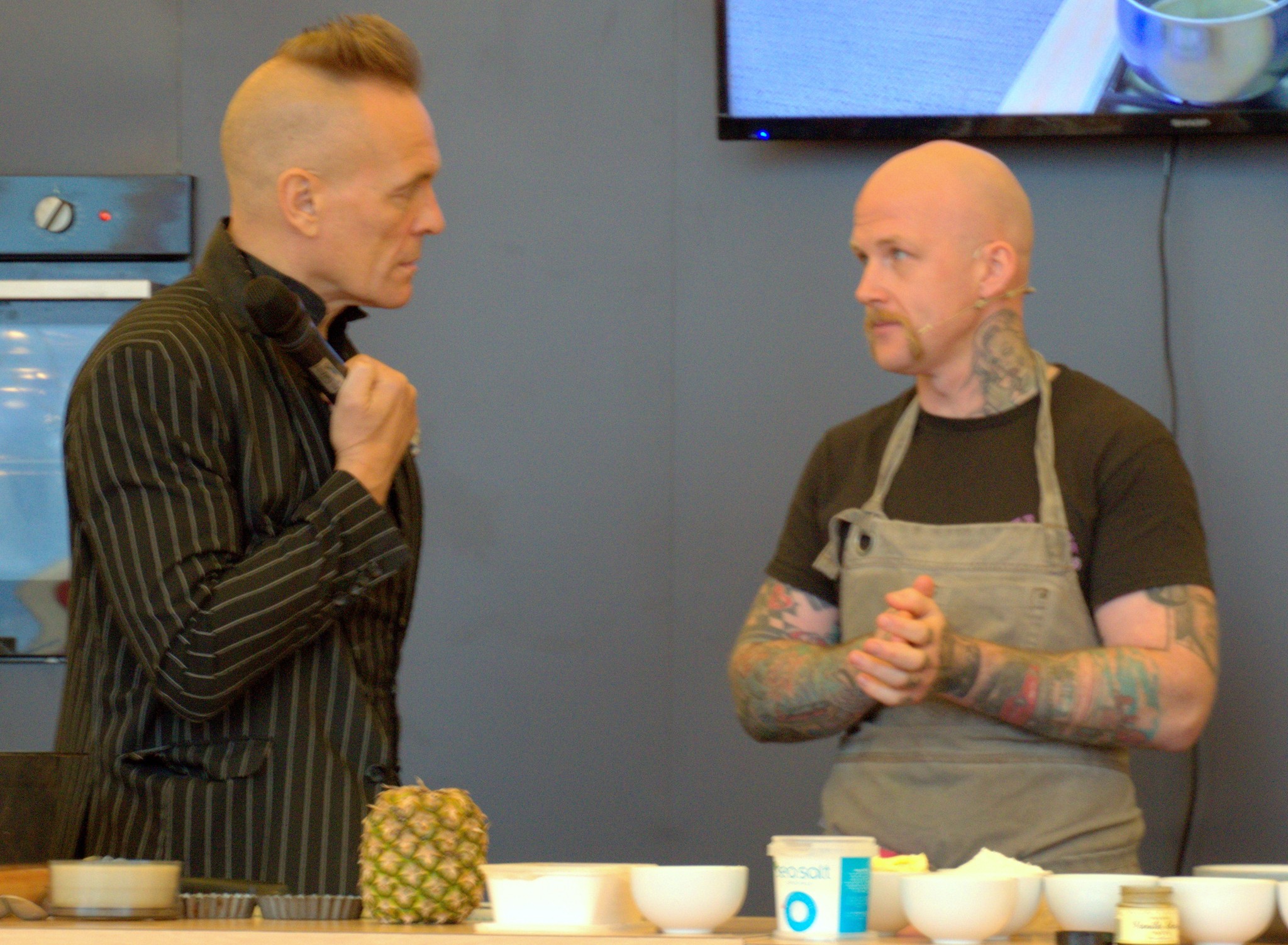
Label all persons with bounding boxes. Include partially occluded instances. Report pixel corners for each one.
[727,136,1220,877]
[46,11,448,901]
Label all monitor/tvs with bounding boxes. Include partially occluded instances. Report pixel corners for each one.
[711,0,1287,147]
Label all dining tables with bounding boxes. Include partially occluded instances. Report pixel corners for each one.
[1,900,1288,945]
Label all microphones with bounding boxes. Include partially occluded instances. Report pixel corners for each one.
[242,276,420,455]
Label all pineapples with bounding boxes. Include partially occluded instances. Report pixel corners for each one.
[356,776,491,924]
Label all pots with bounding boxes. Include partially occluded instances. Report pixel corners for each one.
[1115,0,1288,102]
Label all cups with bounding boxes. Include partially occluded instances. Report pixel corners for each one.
[762,834,874,941]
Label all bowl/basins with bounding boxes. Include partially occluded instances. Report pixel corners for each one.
[475,859,652,933]
[869,866,1288,943]
[2,863,47,906]
[51,855,179,922]
[633,859,748,938]
